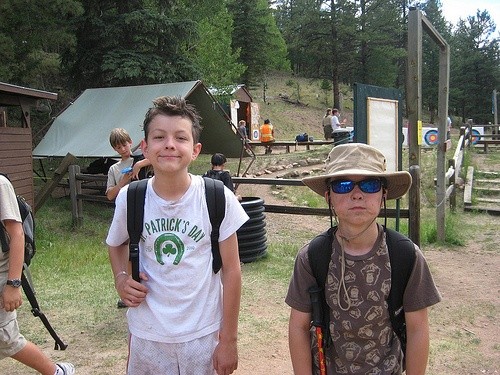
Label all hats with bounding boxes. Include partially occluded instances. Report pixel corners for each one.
[301,142,412,200]
[264,119,270,124]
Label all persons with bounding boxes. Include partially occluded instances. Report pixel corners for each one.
[285,143,441,375]
[322,108,333,141]
[106,98,249,375]
[202,153,234,193]
[236,120,246,137]
[1,174,75,375]
[260,119,275,155]
[447,117,451,140]
[330,109,345,132]
[105,128,154,200]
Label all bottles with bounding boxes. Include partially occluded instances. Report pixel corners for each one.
[304,133,308,140]
[25,244,33,256]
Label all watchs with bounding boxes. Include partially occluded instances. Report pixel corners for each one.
[6,280,22,288]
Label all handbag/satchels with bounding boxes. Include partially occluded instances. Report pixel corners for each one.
[295,133,314,141]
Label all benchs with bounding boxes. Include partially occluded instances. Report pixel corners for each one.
[298,140,335,150]
[476,140,500,153]
[247,140,298,153]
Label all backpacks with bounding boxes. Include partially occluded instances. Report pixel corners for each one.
[0,172,37,273]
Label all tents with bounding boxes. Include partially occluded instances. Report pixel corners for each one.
[31,79,257,159]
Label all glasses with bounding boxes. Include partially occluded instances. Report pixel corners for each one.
[327,178,385,193]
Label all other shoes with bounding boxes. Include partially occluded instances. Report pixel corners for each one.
[40,362,75,375]
[265,148,272,155]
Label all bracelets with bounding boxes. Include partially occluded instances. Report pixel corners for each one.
[114,271,127,279]
